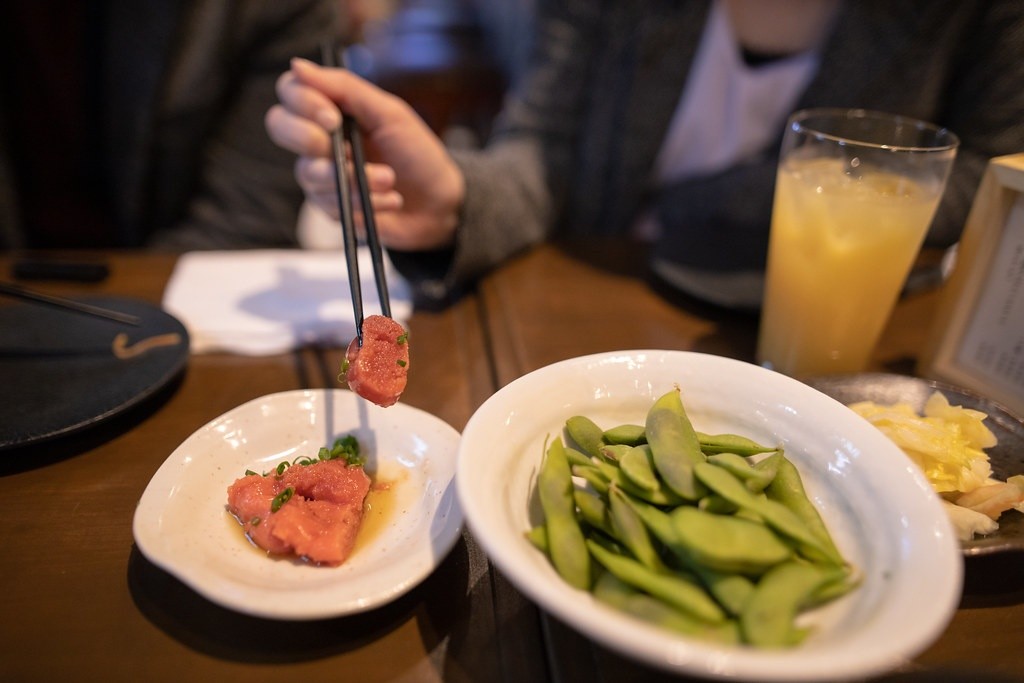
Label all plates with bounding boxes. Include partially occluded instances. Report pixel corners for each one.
[0,293,194,459]
[454,351,967,682]
[793,369,1024,556]
[134,387,469,618]
[639,202,763,309]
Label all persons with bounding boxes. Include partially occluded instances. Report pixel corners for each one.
[263,0,1024,312]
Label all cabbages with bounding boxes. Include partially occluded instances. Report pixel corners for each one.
[844,386,1023,545]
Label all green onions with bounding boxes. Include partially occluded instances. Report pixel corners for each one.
[245,331,408,524]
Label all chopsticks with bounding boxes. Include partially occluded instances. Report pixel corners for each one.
[314,40,396,348]
[0,282,143,326]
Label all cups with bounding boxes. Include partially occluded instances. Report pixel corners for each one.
[756,110,959,383]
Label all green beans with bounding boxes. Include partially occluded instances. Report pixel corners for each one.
[522,386,863,647]
